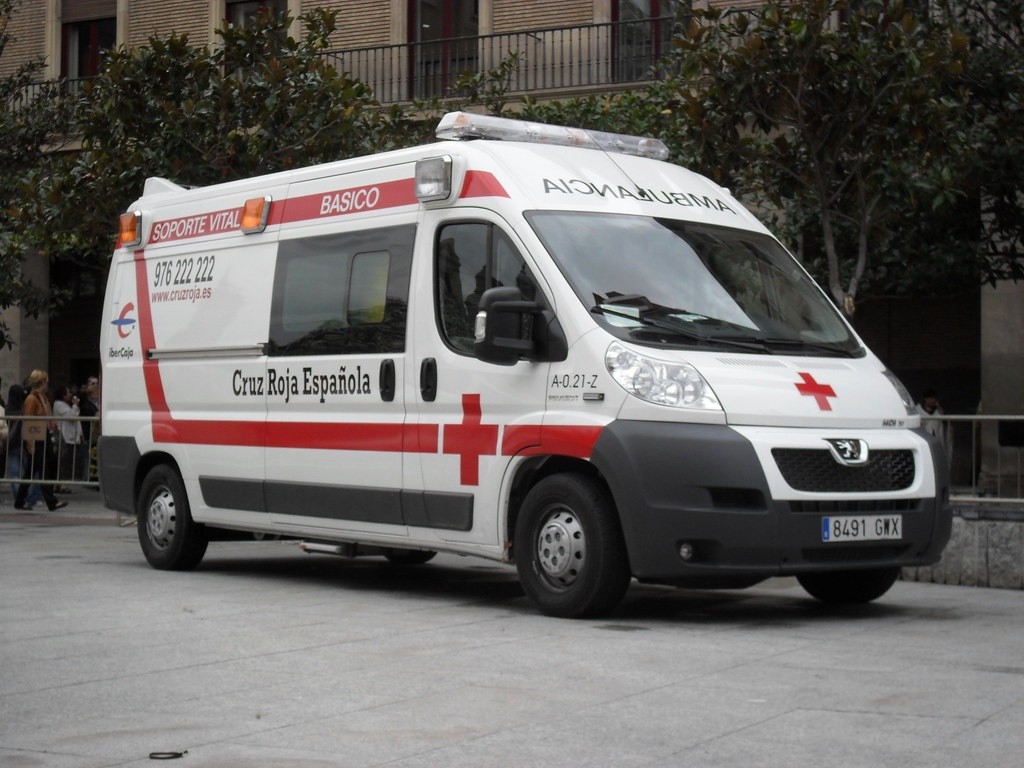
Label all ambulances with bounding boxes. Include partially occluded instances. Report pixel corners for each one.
[95,111,952,621]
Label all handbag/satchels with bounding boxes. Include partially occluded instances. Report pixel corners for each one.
[50,428,71,463]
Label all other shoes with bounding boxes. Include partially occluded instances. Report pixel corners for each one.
[13,503,34,510]
[49,501,68,511]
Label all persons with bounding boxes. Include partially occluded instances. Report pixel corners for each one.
[14,367,69,512]
[916,386,947,458]
[0,375,100,507]
